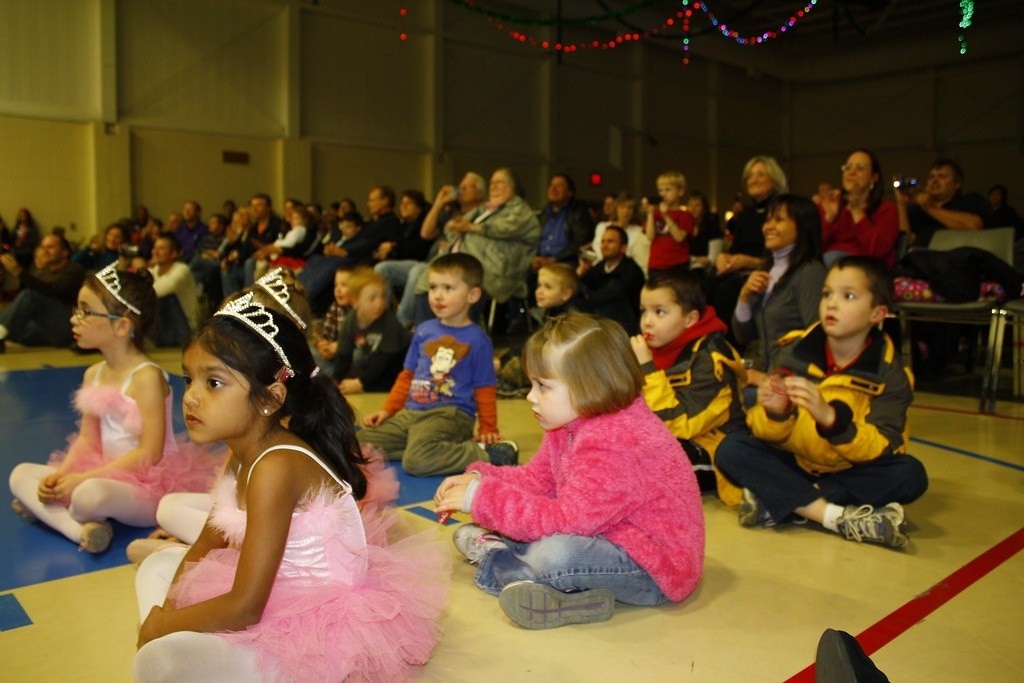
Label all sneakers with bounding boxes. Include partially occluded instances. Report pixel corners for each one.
[452,522,510,568]
[739,487,808,528]
[498,581,616,631]
[837,501,911,550]
[477,439,519,467]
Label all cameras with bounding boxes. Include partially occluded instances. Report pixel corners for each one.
[894,177,917,192]
[121,245,143,258]
[583,248,597,262]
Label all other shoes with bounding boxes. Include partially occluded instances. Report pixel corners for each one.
[815,628,890,683]
[12,499,40,524]
[79,522,112,553]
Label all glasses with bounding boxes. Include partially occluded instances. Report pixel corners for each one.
[841,164,868,172]
[71,306,135,339]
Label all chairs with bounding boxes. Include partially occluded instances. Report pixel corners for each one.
[894,228,1023,412]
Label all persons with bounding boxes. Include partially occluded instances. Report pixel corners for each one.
[495,264,592,400]
[1,166,542,356]
[710,156,823,358]
[580,225,648,339]
[984,183,1024,271]
[816,629,894,683]
[727,194,829,382]
[714,254,929,551]
[891,159,986,389]
[579,188,650,284]
[816,144,901,270]
[646,168,723,291]
[357,252,518,476]
[434,310,705,628]
[525,170,596,310]
[9,260,232,553]
[137,267,454,682]
[628,277,748,508]
[332,264,414,394]
